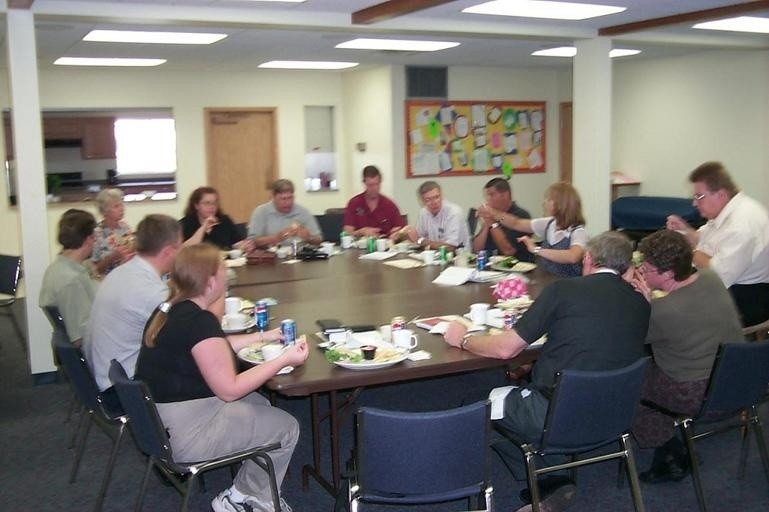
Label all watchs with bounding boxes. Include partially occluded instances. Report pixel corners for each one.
[461,333,474,351]
[535,246,541,254]
[417,237,425,244]
[489,223,501,229]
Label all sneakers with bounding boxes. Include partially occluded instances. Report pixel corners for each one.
[211,488,243,512]
[243,496,293,512]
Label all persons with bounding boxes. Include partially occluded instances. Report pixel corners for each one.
[631,230,746,485]
[475,183,586,277]
[390,182,468,257]
[473,178,542,273]
[248,179,323,250]
[86,215,183,419]
[39,209,95,349]
[444,231,653,512]
[667,162,768,329]
[345,166,403,240]
[179,187,239,259]
[135,244,309,511]
[90,189,136,278]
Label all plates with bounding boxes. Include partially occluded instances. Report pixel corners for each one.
[239,345,289,366]
[330,342,409,370]
[220,314,256,334]
[491,261,536,273]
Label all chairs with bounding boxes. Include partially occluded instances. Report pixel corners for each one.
[40,304,132,508]
[348,400,493,512]
[108,358,285,512]
[616,336,769,512]
[524,356,649,512]
[0,256,29,351]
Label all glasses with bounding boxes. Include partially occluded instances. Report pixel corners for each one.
[641,262,657,275]
[201,201,217,206]
[692,193,705,201]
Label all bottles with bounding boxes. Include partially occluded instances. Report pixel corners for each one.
[280,319,297,347]
[476,251,487,273]
[291,240,297,257]
[392,316,407,344]
[340,231,349,247]
[440,245,447,261]
[505,312,515,331]
[254,301,270,329]
[368,236,375,253]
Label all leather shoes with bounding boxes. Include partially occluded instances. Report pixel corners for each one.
[519,476,570,504]
[515,476,579,512]
[639,453,700,484]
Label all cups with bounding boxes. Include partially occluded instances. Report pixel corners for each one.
[424,251,436,265]
[377,239,389,251]
[341,236,352,249]
[262,344,283,363]
[470,303,489,325]
[231,250,242,258]
[393,330,418,350]
[225,298,240,314]
